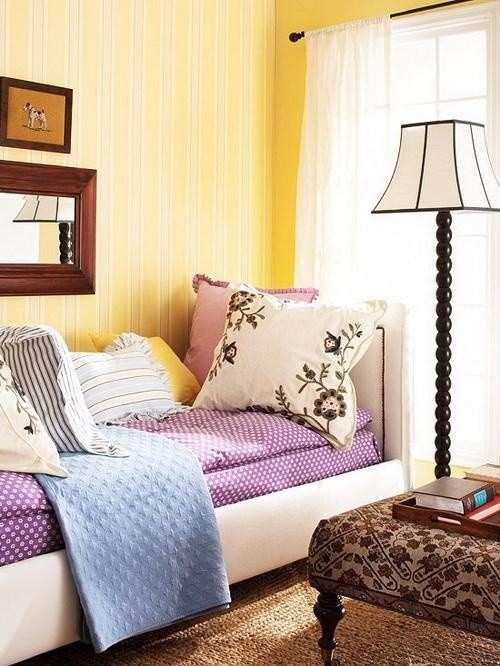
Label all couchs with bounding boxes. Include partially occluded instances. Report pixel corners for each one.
[0,300,410,665]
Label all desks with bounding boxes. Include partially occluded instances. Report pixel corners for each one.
[303,461,500,665]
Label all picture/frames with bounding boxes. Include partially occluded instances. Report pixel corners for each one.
[0,74,73,158]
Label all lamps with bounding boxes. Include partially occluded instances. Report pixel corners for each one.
[11,193,78,265]
[370,120,500,483]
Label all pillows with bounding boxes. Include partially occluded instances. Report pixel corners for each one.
[65,332,186,427]
[2,321,129,462]
[182,273,317,391]
[190,280,385,452]
[1,361,72,480]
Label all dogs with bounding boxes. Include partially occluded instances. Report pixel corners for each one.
[23,102,47,131]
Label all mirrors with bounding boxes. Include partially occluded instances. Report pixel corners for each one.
[0,160,98,297]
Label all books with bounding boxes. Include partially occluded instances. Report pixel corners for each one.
[412,463,500,525]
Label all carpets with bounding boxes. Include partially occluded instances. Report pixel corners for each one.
[90,556,499,664]
